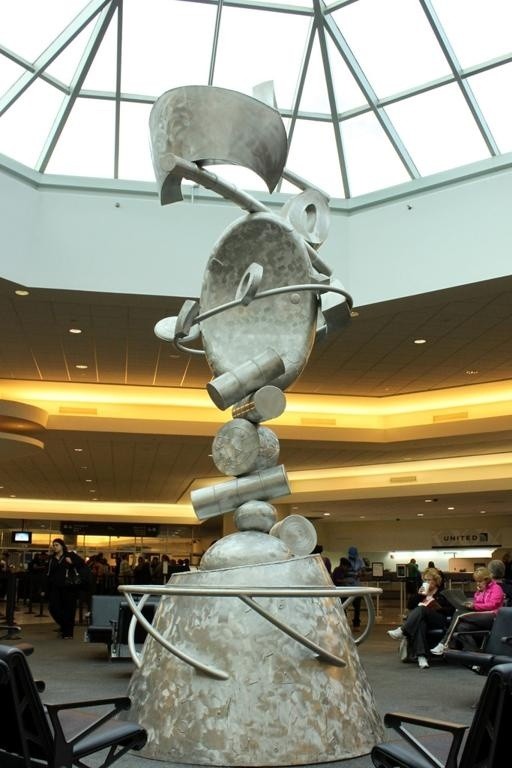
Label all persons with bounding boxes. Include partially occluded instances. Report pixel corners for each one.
[387,555,512,669]
[323,546,366,627]
[0,538,192,640]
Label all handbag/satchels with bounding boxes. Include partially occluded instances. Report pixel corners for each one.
[62,566,82,596]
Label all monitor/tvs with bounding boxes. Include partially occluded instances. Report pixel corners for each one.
[11,531,33,544]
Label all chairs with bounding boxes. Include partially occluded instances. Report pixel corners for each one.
[0,602,155,768]
[372,607,512,768]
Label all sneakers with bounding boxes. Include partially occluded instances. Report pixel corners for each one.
[418,655,429,669]
[386,626,407,641]
[429,642,449,656]
